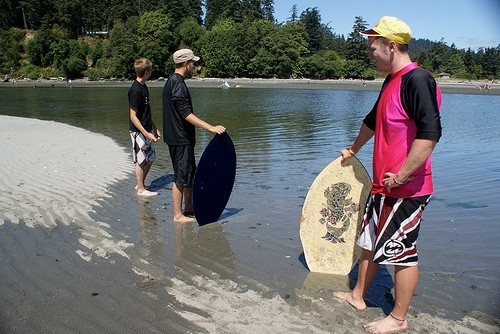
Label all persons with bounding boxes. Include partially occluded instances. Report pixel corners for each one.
[333,16,442,334]
[127,58,161,196]
[162,49,226,223]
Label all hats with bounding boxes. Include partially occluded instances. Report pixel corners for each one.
[359,15,412,44]
[173,49,200,63]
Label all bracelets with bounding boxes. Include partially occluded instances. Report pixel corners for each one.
[349,147,356,155]
[393,173,408,185]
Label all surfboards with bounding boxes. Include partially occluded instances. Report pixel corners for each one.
[192,130,237,226]
[300,155,372,276]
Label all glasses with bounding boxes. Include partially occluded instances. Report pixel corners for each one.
[147,69,154,71]
